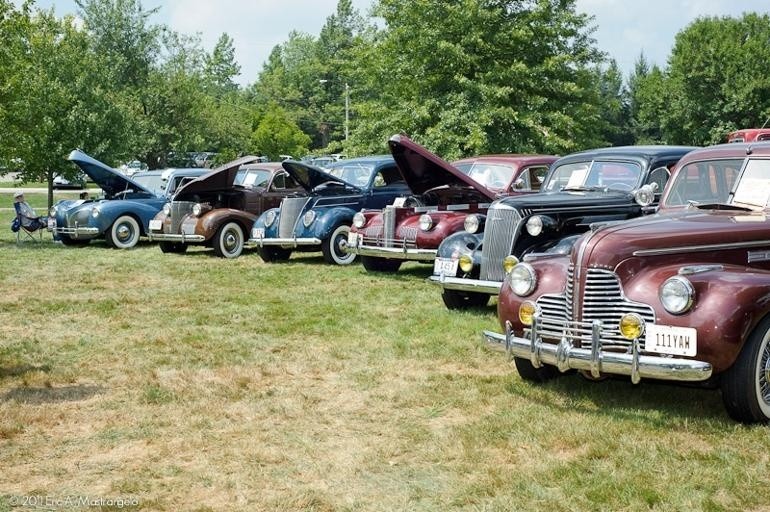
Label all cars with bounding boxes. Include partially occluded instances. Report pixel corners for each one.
[47,128,770,424]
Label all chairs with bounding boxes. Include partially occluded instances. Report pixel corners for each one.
[14,202,49,248]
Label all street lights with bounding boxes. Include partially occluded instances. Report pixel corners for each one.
[318,79,350,153]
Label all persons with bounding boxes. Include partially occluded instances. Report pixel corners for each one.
[81,191,91,199]
[13,190,48,233]
